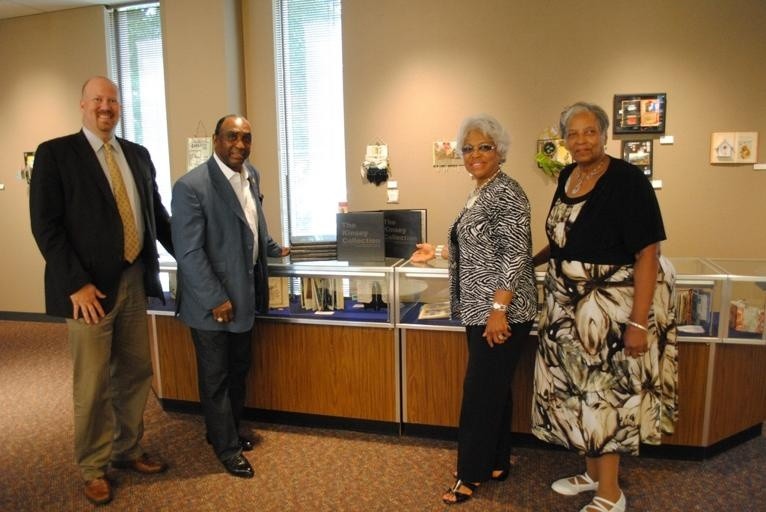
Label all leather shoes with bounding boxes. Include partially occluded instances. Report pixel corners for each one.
[83,475,111,505]
[551,472,598,496]
[579,490,625,512]
[111,454,165,476]
[205,433,254,478]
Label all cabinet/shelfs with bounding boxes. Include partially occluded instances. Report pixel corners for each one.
[147,254,406,436]
[394,257,766,459]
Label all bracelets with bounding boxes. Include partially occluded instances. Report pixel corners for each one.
[623,315,651,336]
[433,242,447,261]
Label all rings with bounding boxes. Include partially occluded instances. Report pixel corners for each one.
[215,314,225,326]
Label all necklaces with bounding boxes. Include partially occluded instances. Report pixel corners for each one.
[568,158,604,195]
[470,169,502,196]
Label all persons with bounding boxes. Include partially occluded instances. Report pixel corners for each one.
[411,111,536,505]
[533,103,676,512]
[171,113,292,478]
[31,78,173,507]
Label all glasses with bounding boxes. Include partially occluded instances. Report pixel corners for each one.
[461,145,495,153]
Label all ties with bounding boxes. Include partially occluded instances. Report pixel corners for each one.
[102,143,140,264]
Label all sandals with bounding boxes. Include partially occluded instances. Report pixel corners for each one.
[442,470,508,503]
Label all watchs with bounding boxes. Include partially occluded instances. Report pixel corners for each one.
[489,299,509,314]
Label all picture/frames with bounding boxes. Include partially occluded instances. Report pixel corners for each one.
[613,93,666,133]
[621,139,653,178]
[24,152,36,185]
[537,139,573,168]
